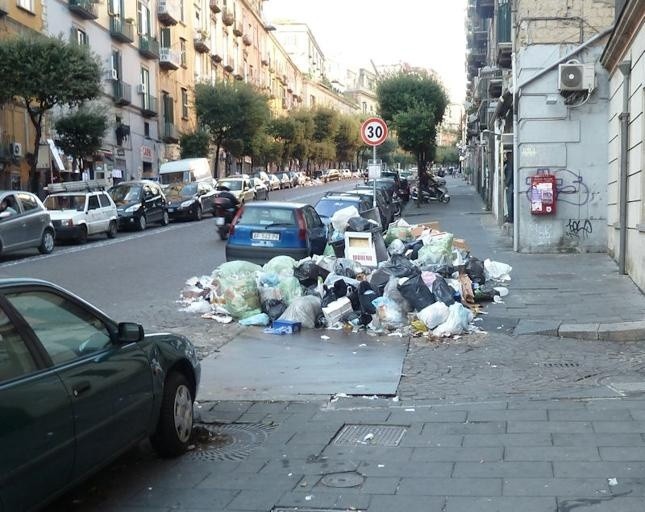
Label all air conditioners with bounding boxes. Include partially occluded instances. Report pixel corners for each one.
[558,63,595,91]
[108,69,117,80]
[138,84,145,94]
[10,143,22,156]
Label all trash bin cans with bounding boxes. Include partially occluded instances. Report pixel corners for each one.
[328,225,388,265]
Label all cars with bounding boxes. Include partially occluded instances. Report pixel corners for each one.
[107,180,170,231]
[225,201,328,265]
[1,278,200,512]
[314,169,416,238]
[42,190,119,245]
[215,172,306,204]
[165,182,219,221]
[0,190,57,259]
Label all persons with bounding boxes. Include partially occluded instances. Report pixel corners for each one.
[214,183,239,223]
[416,165,435,201]
[436,166,445,178]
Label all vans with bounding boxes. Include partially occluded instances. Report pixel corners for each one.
[159,158,215,190]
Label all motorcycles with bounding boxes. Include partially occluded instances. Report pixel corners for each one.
[410,184,450,204]
[215,198,238,239]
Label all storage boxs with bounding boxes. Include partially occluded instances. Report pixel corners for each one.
[344,242,377,267]
[322,296,353,327]
[399,225,425,236]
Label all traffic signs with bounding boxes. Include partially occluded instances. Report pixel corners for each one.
[361,118,388,146]
